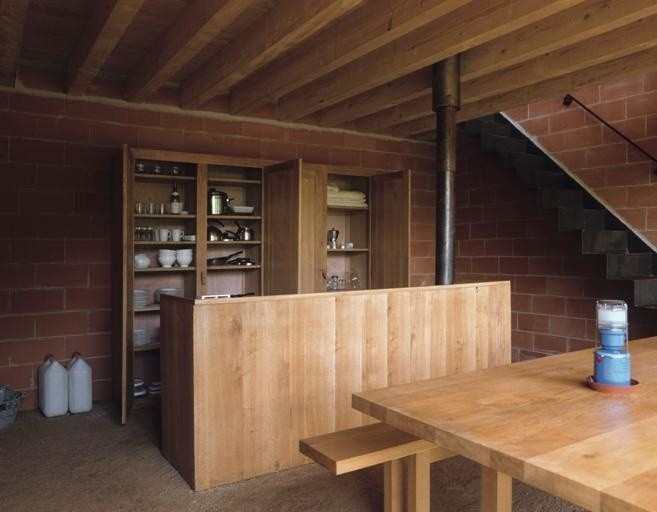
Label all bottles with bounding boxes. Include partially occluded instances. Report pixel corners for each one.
[168,180,180,215]
[327,273,359,291]
[339,242,345,250]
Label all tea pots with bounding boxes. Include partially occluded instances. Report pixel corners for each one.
[234,220,254,241]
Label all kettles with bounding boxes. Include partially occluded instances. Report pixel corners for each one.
[207,219,224,242]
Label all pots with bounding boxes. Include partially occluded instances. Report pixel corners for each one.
[207,250,242,265]
[206,187,233,215]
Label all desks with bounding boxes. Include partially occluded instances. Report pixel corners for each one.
[350,333,657,512]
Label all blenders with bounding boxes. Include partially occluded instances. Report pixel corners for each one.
[326,226,339,249]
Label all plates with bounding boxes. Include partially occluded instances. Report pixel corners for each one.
[131,287,181,309]
[133,328,151,348]
[226,205,254,214]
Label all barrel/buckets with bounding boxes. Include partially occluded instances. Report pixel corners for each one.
[0,381,30,427]
[38,353,70,417]
[68,350,93,412]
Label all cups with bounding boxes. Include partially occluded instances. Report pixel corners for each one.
[135,161,180,175]
[133,226,184,243]
[134,200,165,214]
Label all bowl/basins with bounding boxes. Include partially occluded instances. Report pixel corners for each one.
[344,242,353,250]
[133,248,192,269]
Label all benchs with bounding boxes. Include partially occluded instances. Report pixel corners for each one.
[292,415,458,512]
